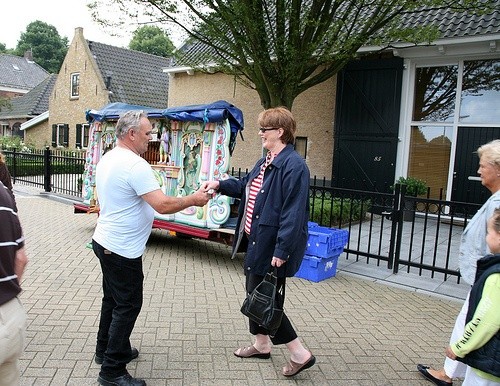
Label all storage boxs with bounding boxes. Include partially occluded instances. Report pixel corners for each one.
[304,221,349,259]
[293,255,340,283]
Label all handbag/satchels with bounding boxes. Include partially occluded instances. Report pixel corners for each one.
[240,262,284,331]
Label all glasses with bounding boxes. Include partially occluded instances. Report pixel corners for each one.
[259,127,279,133]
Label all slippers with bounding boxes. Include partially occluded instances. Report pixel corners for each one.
[282,355,315,377]
[233,345,270,359]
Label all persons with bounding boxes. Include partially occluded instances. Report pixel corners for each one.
[92,110,211,386]
[200,107,317,377]
[0,153,18,214]
[184,151,198,186]
[417,140,500,386]
[157,125,169,165]
[446,208,500,386]
[0,181,28,386]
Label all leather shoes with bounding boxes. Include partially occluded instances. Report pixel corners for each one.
[97,370,146,386]
[95,347,139,364]
[417,363,453,386]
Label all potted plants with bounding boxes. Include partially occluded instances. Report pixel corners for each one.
[390,175,427,220]
